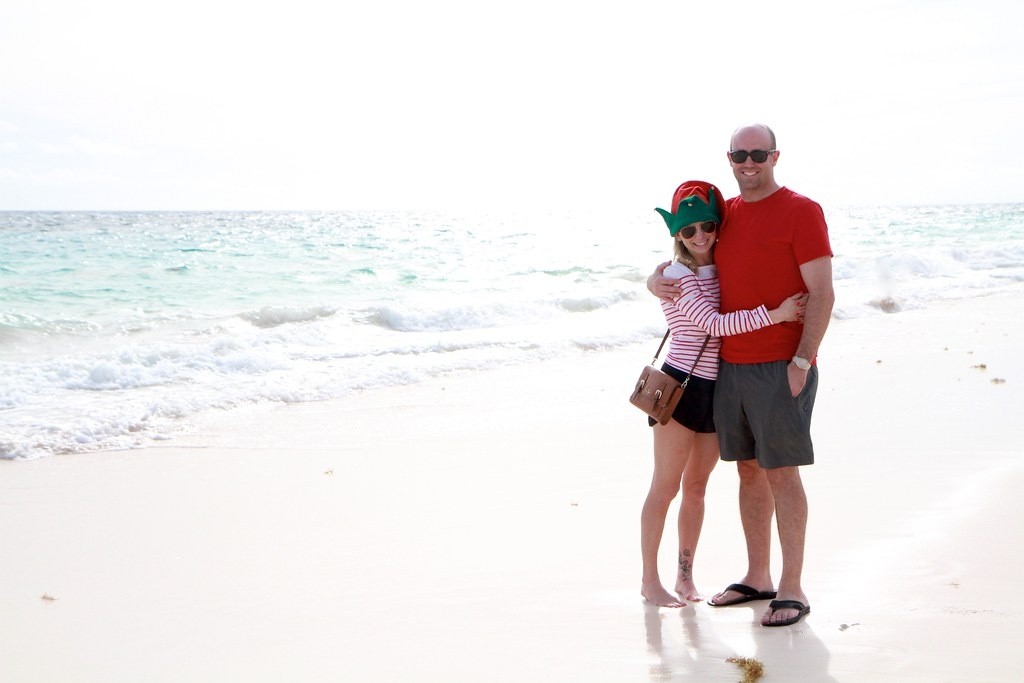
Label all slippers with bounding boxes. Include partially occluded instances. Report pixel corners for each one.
[706,582,778,606]
[761,599,810,626]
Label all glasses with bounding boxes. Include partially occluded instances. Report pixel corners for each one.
[678,220,716,239]
[729,149,775,163]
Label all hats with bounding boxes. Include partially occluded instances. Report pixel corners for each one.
[653,180,727,236]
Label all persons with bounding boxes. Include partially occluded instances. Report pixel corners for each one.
[646,122,836,626]
[639,124,811,626]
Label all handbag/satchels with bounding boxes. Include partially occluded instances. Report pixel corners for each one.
[628,364,684,426]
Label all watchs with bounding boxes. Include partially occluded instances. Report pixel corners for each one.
[789,356,814,372]
